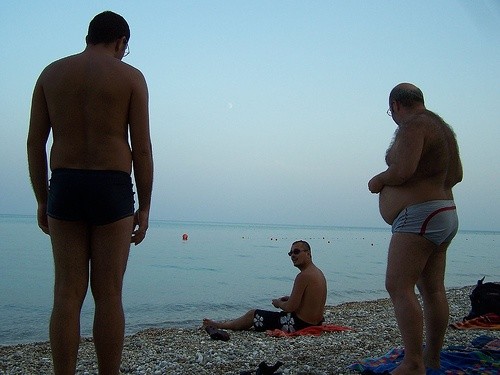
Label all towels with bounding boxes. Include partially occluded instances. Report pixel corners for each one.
[266,324,353,339]
[345,335,499,375]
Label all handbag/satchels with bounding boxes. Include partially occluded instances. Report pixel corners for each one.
[463,276,500,322]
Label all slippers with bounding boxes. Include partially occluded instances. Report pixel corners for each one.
[205,325,231,341]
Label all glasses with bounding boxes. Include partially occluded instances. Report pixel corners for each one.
[288,249,307,256]
[122,43,131,57]
[386,105,391,116]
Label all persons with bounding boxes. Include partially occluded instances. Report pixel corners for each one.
[26,10,153,375]
[202,241,327,334]
[368,82,463,375]
